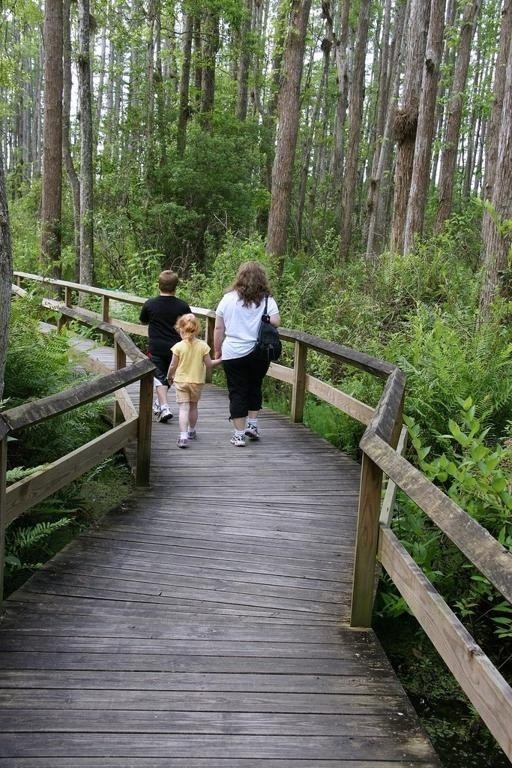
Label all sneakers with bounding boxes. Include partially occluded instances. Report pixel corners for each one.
[153,402,172,422]
[245,423,260,439]
[177,430,195,447]
[230,435,245,446]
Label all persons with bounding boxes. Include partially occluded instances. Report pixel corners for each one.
[214,261,281,447]
[139,270,192,422]
[166,313,222,448]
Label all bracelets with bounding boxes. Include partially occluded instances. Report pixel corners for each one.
[166,376,172,380]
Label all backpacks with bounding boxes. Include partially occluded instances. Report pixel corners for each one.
[255,296,282,361]
